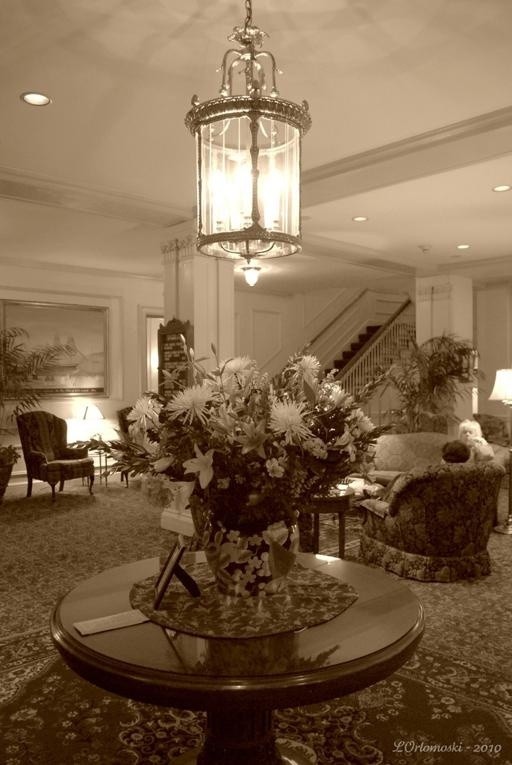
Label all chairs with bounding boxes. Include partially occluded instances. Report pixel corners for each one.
[358,467,506,584]
[16,411,96,499]
[473,412,510,527]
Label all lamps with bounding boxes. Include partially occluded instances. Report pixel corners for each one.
[185,0,310,290]
[488,368,512,536]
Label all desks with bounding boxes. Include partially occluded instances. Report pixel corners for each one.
[312,484,355,560]
[50,551,425,765]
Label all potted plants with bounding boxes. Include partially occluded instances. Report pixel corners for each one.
[0,326,84,503]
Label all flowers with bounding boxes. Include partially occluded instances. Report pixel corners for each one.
[65,334,394,496]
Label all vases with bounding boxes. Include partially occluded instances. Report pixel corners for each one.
[187,494,302,597]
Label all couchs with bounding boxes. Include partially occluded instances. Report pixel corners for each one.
[351,430,510,483]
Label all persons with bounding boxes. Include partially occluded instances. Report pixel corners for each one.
[441,440,469,463]
[458,418,496,462]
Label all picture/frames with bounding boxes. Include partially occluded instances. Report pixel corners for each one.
[0,299,112,398]
[153,534,201,609]
